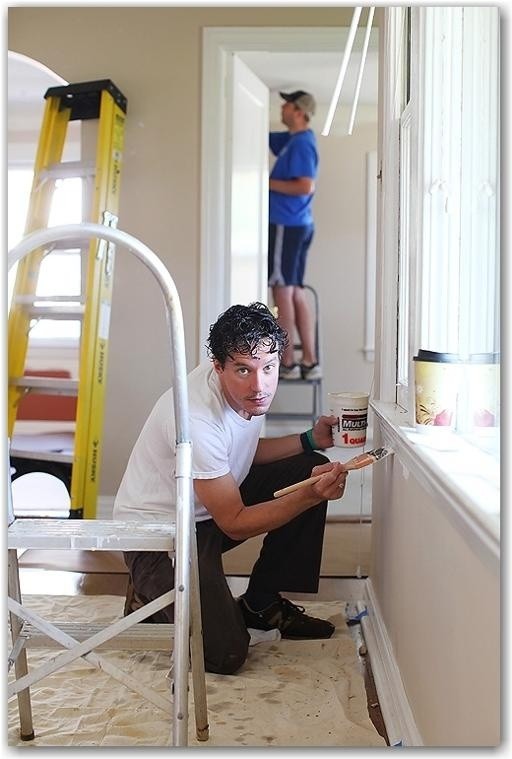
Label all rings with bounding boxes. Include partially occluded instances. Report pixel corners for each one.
[338,482,345,489]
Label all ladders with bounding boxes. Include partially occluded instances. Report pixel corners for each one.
[7,78,128,521]
[265,284,323,429]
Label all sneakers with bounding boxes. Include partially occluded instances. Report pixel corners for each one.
[278,360,303,381]
[297,361,323,382]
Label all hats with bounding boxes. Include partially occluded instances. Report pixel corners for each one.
[277,91,317,121]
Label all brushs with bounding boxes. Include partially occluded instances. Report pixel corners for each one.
[274,446,395,498]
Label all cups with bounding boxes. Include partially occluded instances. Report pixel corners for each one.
[466,352,500,428]
[327,391,372,449]
[412,348,462,434]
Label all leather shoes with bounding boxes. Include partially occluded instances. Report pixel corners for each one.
[235,596,336,640]
[122,574,152,626]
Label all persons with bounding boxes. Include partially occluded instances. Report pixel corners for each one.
[265,86,323,383]
[108,297,351,678]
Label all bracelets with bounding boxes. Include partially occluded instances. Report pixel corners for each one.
[298,428,320,453]
